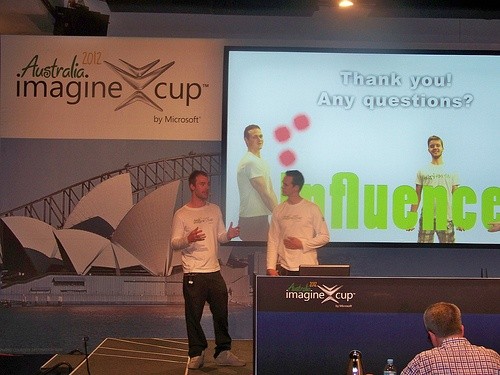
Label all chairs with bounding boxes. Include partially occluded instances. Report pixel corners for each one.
[299,264,351,277]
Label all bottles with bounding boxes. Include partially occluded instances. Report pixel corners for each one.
[383,359,397,375]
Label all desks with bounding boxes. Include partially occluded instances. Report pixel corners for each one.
[252,275,500,375]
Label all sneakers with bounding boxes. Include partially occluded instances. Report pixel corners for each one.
[189,350,205,368]
[215,350,248,366]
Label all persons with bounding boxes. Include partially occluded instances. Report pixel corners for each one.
[405,134,465,243]
[399,302,500,375]
[266,170,330,276]
[487,222,500,232]
[170,170,247,369]
[237,125,278,241]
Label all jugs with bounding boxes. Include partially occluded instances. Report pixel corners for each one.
[346,350,364,375]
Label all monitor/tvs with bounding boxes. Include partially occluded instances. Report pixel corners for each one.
[299,265,351,276]
